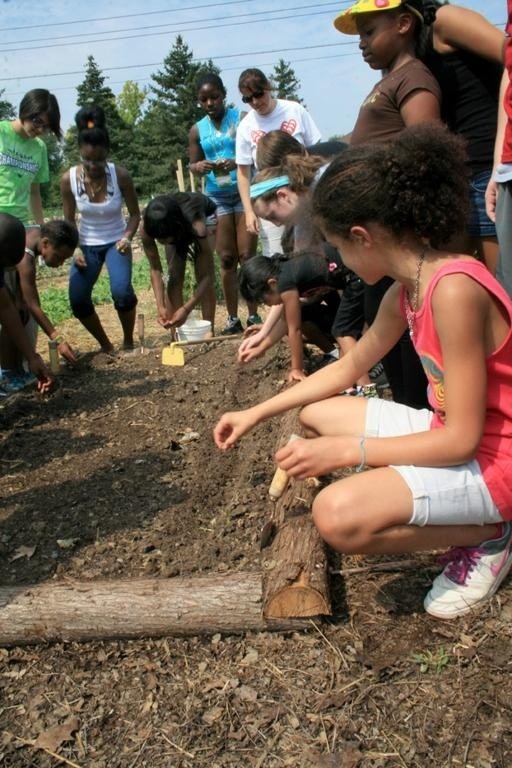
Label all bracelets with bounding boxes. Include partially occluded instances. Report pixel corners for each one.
[183,307,191,314]
[356,435,368,472]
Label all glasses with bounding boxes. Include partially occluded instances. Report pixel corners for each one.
[77,152,108,170]
[29,115,54,134]
[242,84,268,104]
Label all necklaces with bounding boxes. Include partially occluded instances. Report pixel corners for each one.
[84,169,107,199]
[402,241,433,343]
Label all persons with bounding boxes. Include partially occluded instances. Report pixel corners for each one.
[187,72,265,335]
[140,190,221,344]
[212,118,512,621]
[331,1,443,149]
[405,0,504,279]
[5,219,82,368]
[235,68,324,259]
[254,131,401,371]
[237,251,380,400]
[484,0,512,300]
[248,153,396,390]
[0,211,56,398]
[60,105,143,353]
[0,88,63,362]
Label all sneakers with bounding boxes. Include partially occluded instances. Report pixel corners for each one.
[247,314,262,327]
[221,315,242,334]
[423,522,512,620]
[20,370,37,386]
[1,369,18,391]
[355,362,391,388]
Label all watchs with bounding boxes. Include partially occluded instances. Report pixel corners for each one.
[54,335,64,347]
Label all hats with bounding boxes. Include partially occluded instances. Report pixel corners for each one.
[333,0,424,36]
